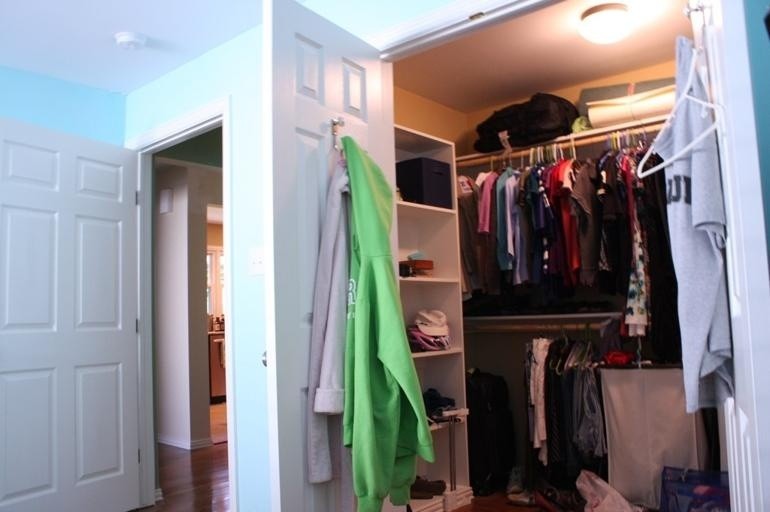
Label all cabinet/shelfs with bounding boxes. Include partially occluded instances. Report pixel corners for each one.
[393,122,476,512]
[208,331,226,405]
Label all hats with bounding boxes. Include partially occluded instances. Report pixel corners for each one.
[412,307,450,338]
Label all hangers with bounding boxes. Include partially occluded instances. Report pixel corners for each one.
[548,321,608,376]
[636,49,724,182]
[486,123,653,171]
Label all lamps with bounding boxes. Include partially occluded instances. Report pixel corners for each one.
[572,4,641,44]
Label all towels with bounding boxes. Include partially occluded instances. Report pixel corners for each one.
[219,339,226,369]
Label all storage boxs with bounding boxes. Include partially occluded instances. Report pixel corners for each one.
[394,155,454,210]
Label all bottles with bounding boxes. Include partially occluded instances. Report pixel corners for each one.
[207,313,225,331]
